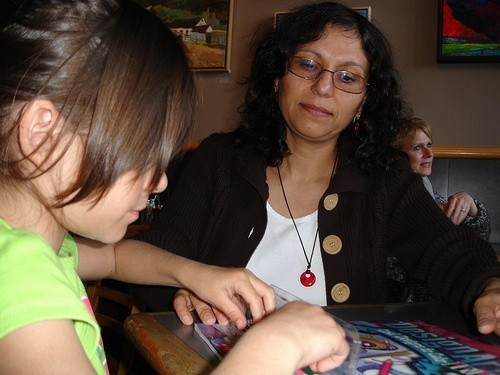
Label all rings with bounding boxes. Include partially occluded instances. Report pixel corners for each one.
[461,208,468,213]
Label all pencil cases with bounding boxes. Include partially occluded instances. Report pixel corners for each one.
[232,282,362,375]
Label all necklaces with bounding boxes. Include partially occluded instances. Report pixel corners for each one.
[275,151,339,288]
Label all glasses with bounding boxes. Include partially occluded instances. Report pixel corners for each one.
[287,56,370,95]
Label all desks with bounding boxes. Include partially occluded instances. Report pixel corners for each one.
[123,313,500,375]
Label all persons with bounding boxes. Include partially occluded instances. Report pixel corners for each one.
[0,0,350,375]
[397,118,490,240]
[150,0,500,336]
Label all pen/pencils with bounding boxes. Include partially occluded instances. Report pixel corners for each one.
[377,358,393,375]
[243,313,319,375]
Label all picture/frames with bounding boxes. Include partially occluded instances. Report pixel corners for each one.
[272,6,373,31]
[140,0,235,73]
